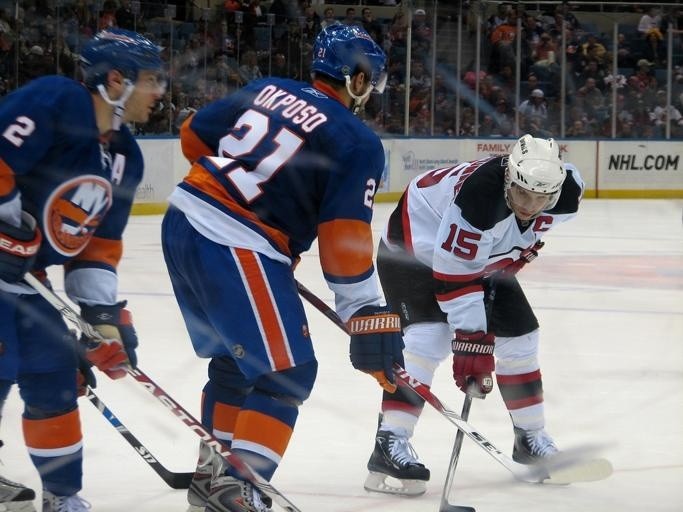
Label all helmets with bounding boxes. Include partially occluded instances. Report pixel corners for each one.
[502,133,567,220]
[78,27,167,89]
[311,21,389,96]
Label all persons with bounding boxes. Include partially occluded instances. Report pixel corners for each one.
[161,27,405,512]
[0,29,166,512]
[367,134,583,480]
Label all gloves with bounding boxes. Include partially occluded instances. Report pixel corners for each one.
[81,299,141,379]
[346,307,408,396]
[451,327,497,401]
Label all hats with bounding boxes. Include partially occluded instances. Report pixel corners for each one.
[529,89,545,98]
[637,58,656,67]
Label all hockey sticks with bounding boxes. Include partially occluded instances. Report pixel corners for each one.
[83,385,195,489]
[440,394,475,512]
[297,281,613,483]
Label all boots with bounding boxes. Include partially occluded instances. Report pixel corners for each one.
[512,424,562,469]
[188,443,274,512]
[367,413,430,481]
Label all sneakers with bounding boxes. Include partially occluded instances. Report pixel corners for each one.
[0,475,36,503]
